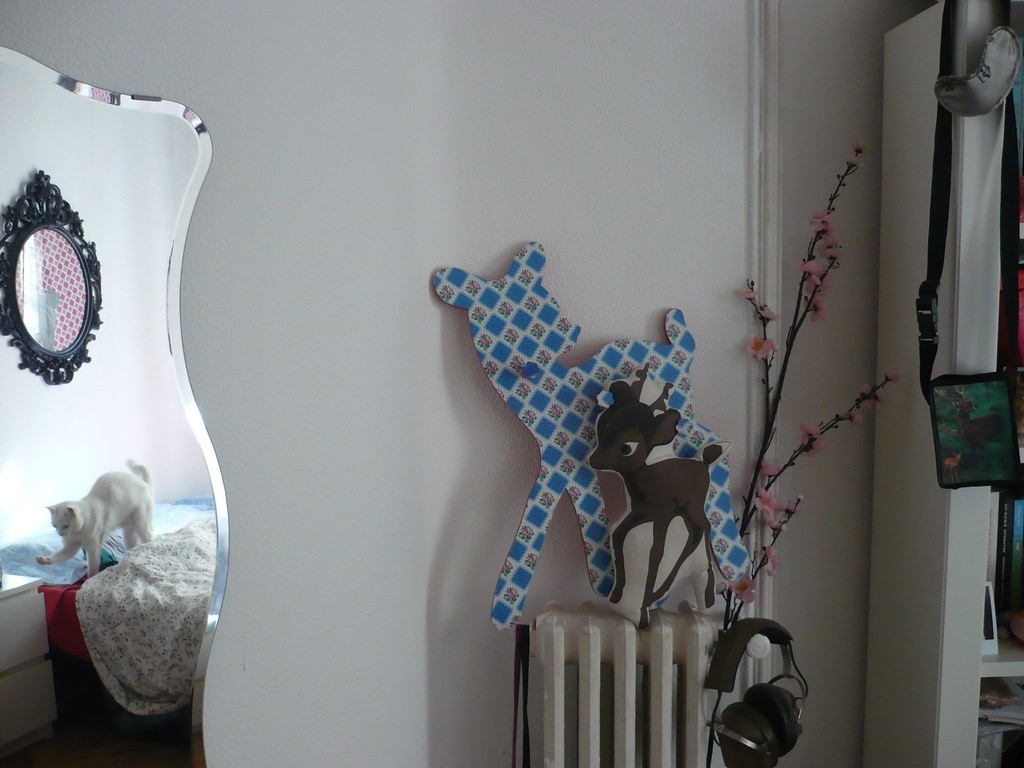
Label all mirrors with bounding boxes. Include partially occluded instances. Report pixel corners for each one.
[0,169,104,384]
[0,46,231,768]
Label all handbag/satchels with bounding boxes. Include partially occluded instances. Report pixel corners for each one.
[930,370,1023,487]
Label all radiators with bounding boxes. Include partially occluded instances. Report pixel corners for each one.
[529,600,718,768]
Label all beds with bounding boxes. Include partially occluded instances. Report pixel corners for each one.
[0,498,218,717]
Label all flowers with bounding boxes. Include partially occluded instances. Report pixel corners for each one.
[715,131,904,629]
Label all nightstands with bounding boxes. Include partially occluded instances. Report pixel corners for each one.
[0,574,59,756]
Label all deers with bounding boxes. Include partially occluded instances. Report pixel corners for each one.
[587,364,730,627]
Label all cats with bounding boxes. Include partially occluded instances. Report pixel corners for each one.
[35,459,154,578]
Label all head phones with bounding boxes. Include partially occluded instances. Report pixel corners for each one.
[705,617,808,768]
